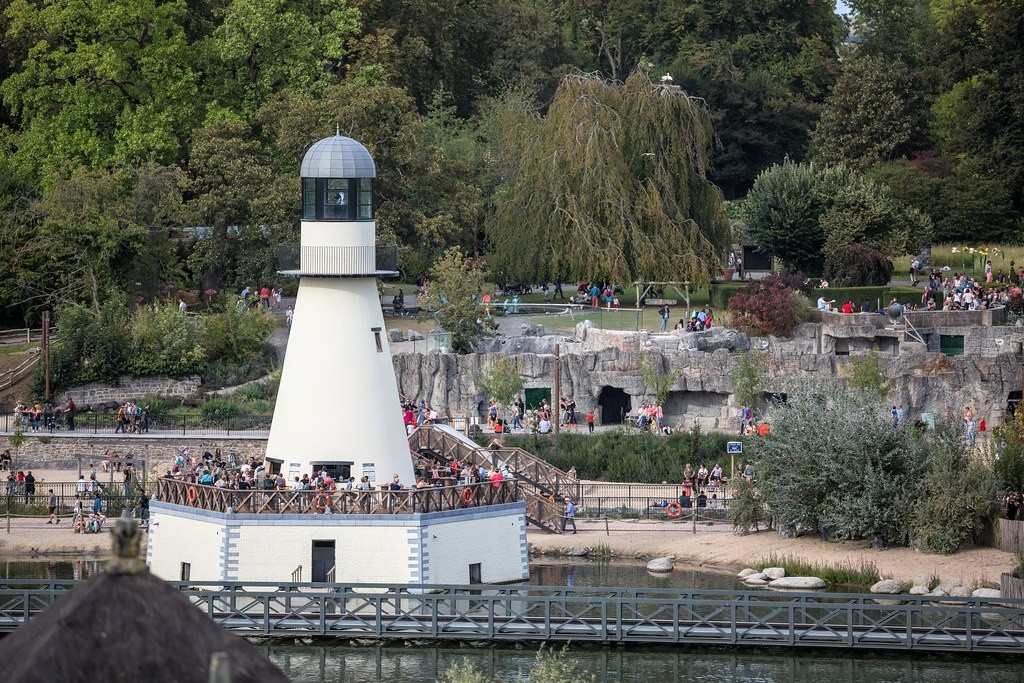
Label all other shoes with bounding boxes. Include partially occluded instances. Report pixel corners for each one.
[32,430,38,433]
[46,521,52,524]
[56,519,61,525]
[573,532,576,534]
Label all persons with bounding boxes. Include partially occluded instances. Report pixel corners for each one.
[566,464,576,484]
[561,397,596,433]
[392,281,619,336]
[164,447,287,511]
[487,396,553,433]
[562,498,577,534]
[537,489,556,526]
[401,399,438,435]
[0,449,149,534]
[115,402,150,433]
[736,257,1024,314]
[293,462,371,513]
[740,404,770,436]
[180,286,293,325]
[892,406,987,447]
[638,403,664,435]
[16,397,75,433]
[488,439,502,468]
[378,457,510,510]
[659,303,713,332]
[679,461,752,508]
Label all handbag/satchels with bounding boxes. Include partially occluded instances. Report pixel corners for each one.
[71,406,76,412]
[596,288,600,298]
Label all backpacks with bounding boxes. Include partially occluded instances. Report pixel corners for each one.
[607,289,611,297]
[517,407,522,415]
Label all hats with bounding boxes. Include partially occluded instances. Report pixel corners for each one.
[966,285,969,288]
[564,498,570,502]
[540,491,544,494]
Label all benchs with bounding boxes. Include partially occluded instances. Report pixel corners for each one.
[381,304,404,316]
[626,412,638,424]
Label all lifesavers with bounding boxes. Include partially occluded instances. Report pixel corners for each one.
[666,502,682,518]
[314,494,330,510]
[462,487,474,504]
[187,486,198,501]
